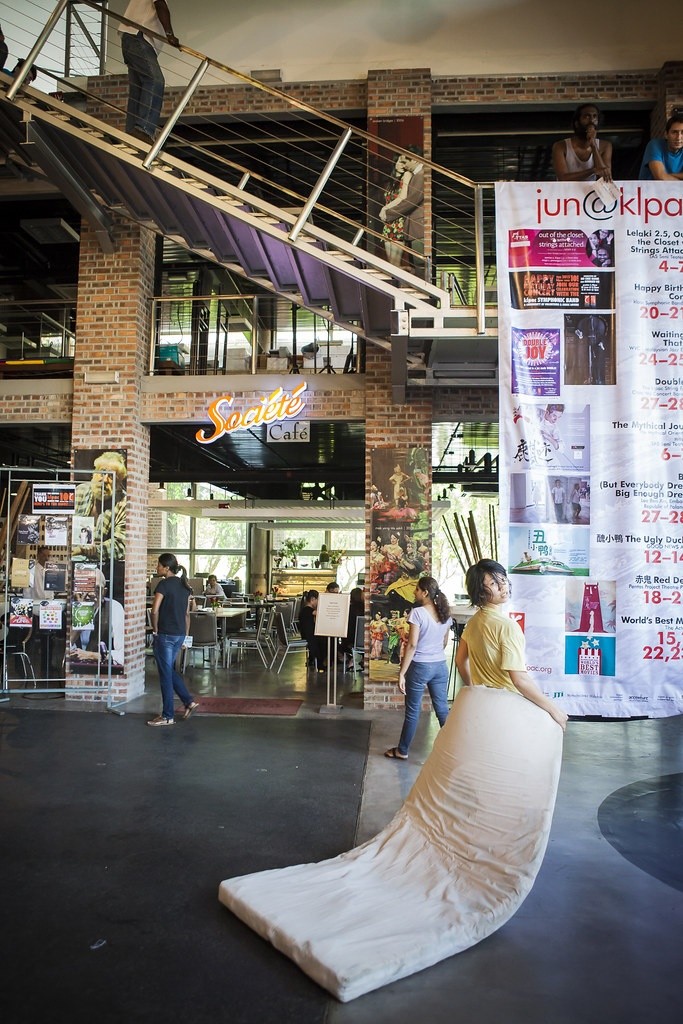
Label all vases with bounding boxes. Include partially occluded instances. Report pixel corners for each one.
[293,553,300,568]
[272,592,278,599]
[254,596,260,602]
[211,602,218,610]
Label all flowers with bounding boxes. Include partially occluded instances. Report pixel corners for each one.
[210,597,219,603]
[286,536,308,552]
[271,586,280,591]
[251,590,262,596]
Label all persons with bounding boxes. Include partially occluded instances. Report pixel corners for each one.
[455,559,569,732]
[71,451,127,561]
[638,108,683,180]
[514,404,565,469]
[3,58,37,85]
[575,316,605,384]
[552,104,613,183]
[23,547,55,674]
[202,574,227,601]
[300,582,364,672]
[146,553,199,727]
[379,145,426,279]
[589,229,613,267]
[117,0,179,151]
[79,526,91,544]
[551,480,590,522]
[384,577,453,760]
[0,613,23,702]
[0,24,8,71]
[371,463,430,670]
[70,568,124,665]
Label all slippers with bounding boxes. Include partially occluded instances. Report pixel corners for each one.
[384,748,409,760]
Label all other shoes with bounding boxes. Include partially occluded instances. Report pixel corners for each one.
[147,715,176,726]
[182,701,200,719]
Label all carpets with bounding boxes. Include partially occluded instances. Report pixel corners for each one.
[174,696,304,717]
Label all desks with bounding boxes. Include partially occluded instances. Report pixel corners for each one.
[196,605,251,639]
[224,600,288,629]
[260,595,297,643]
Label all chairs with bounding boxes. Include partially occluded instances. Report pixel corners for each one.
[146,591,364,677]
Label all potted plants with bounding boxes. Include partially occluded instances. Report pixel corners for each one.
[330,549,349,568]
[320,544,329,569]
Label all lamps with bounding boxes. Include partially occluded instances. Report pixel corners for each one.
[209,483,213,500]
[437,422,478,501]
[158,482,166,492]
[186,483,194,501]
